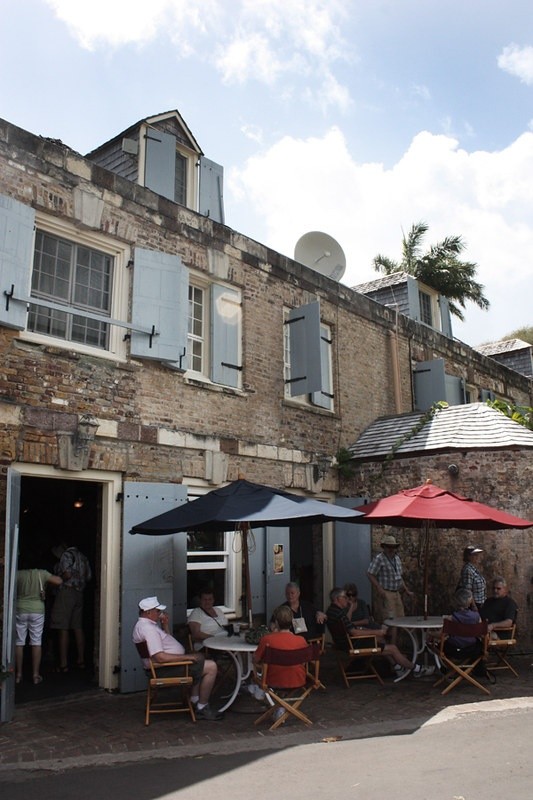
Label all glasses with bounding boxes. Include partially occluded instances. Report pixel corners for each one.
[346,592,357,597]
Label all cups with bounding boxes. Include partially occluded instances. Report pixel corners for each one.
[158,612,169,623]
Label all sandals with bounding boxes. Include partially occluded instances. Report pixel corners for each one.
[71,662,86,670]
[15,673,23,683]
[33,675,43,684]
[47,665,69,674]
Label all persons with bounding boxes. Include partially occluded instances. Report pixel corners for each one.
[434,545,516,679]
[54,526,92,673]
[270,582,327,644]
[187,589,265,701]
[367,536,414,643]
[253,606,308,724]
[132,596,224,720]
[326,583,436,683]
[15,552,63,685]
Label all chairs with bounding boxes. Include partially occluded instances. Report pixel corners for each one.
[134,617,517,732]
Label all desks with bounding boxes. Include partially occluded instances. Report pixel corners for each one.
[384,615,452,684]
[202,634,275,713]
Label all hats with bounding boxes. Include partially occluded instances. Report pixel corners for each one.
[138,596,167,611]
[380,536,400,547]
[464,546,483,554]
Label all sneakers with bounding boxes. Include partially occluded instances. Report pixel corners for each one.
[255,689,266,700]
[195,701,223,720]
[240,685,258,694]
[414,665,436,678]
[393,667,411,683]
[272,707,285,723]
[191,695,199,707]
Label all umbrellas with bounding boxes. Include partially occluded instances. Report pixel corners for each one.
[351,478,533,666]
[128,474,366,685]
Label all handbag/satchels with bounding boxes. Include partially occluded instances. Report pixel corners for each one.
[292,618,308,634]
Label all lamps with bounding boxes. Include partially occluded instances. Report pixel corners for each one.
[75,411,100,457]
[313,452,333,484]
